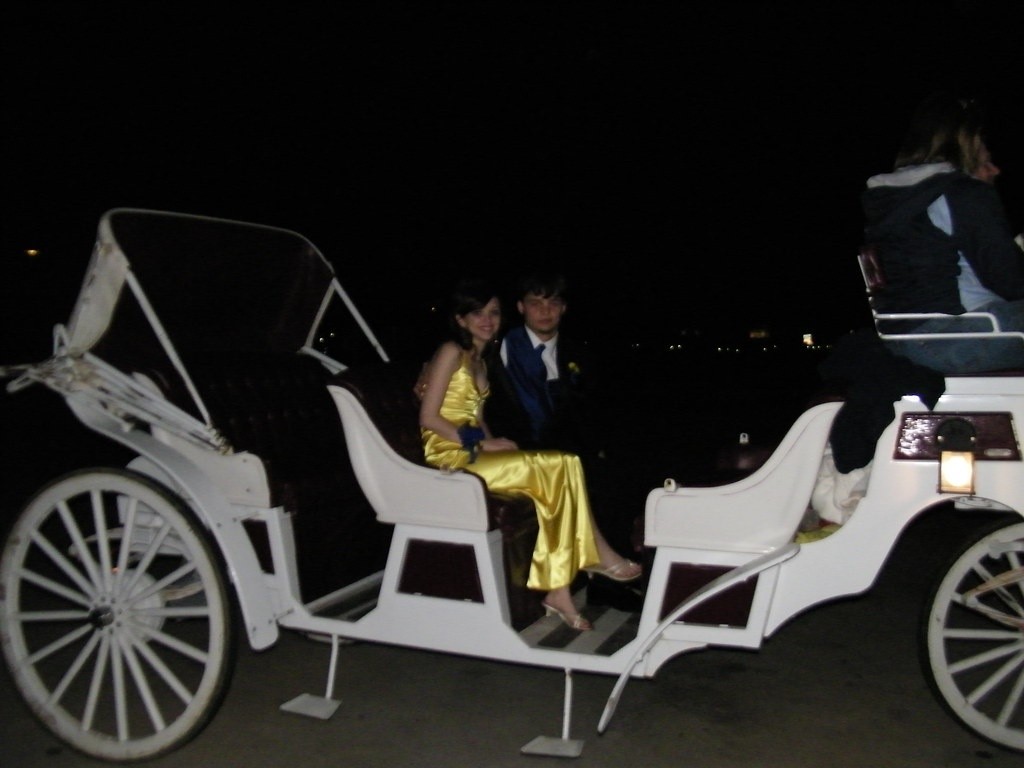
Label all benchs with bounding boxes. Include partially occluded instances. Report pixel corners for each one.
[637,401,846,641]
[326,362,541,629]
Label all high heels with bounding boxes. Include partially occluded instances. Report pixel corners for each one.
[580,559,642,581]
[542,601,592,631]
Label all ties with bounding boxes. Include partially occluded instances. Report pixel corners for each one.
[534,345,547,382]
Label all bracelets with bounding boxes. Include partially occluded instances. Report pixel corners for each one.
[458,421,485,464]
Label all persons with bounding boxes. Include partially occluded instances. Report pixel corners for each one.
[483,276,644,616]
[414,276,644,632]
[852,92,1024,377]
